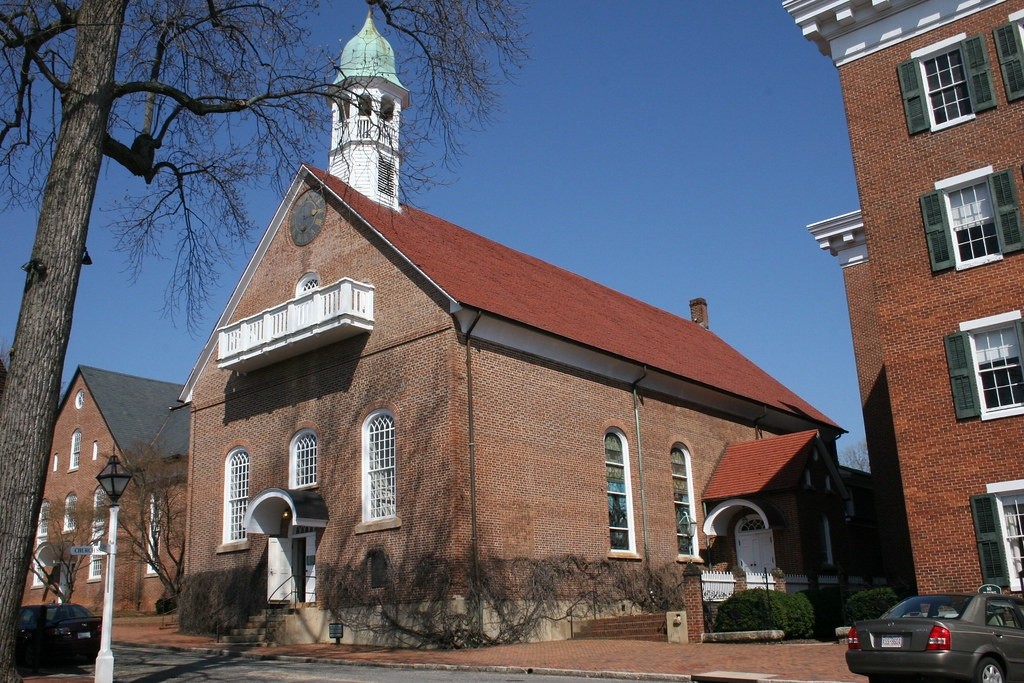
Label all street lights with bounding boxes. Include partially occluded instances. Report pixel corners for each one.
[95,447,133,683]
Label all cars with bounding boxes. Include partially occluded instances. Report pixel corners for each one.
[846,592,1024,683]
[15,604,102,669]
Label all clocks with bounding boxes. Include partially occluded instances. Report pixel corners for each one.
[289,188,327,247]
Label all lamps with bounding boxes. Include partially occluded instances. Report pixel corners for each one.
[742,516,748,523]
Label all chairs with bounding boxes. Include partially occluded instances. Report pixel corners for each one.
[987,614,1003,626]
